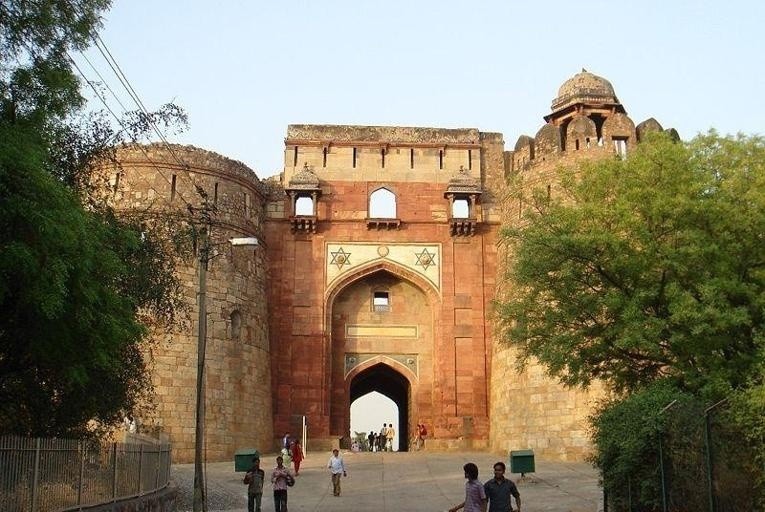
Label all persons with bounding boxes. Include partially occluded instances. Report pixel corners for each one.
[327,449,347,497]
[244,457,265,512]
[484,461,521,512]
[368,423,395,451]
[283,431,304,476]
[271,456,295,512]
[416,424,427,448]
[449,463,487,512]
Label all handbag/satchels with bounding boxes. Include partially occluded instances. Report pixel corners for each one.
[286,474,296,488]
